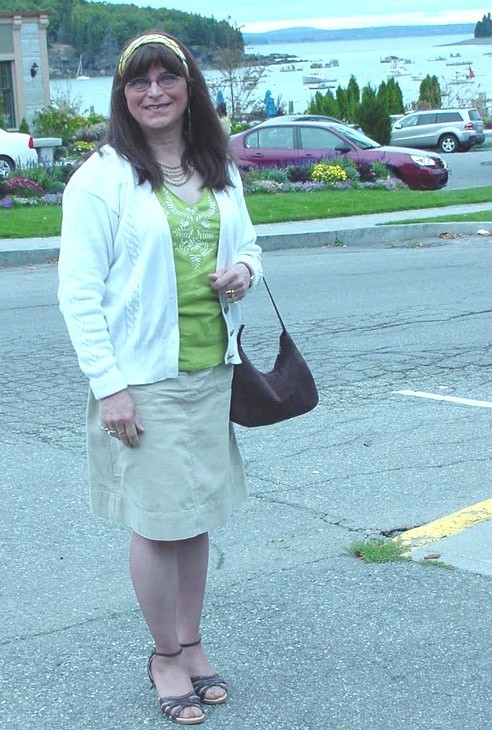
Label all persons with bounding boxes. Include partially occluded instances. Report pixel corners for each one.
[59,28,263,724]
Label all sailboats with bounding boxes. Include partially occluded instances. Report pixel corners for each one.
[75,55,90,80]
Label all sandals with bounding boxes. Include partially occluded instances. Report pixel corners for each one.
[177,635,228,703]
[147,646,206,725]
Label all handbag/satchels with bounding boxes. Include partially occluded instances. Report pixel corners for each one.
[225,254,318,427]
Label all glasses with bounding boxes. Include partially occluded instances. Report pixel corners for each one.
[126,72,183,92]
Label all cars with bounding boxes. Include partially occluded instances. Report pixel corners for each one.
[0,127,39,182]
[228,115,450,191]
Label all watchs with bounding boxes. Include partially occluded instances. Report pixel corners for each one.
[241,262,255,288]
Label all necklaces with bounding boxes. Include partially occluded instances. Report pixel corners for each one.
[157,161,195,187]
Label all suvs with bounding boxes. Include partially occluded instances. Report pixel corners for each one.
[389,108,486,152]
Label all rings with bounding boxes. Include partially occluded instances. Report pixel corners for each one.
[225,289,234,294]
[108,430,116,435]
[103,427,109,431]
[232,294,235,297]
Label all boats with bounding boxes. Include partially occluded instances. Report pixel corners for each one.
[379,53,477,98]
[282,59,339,91]
